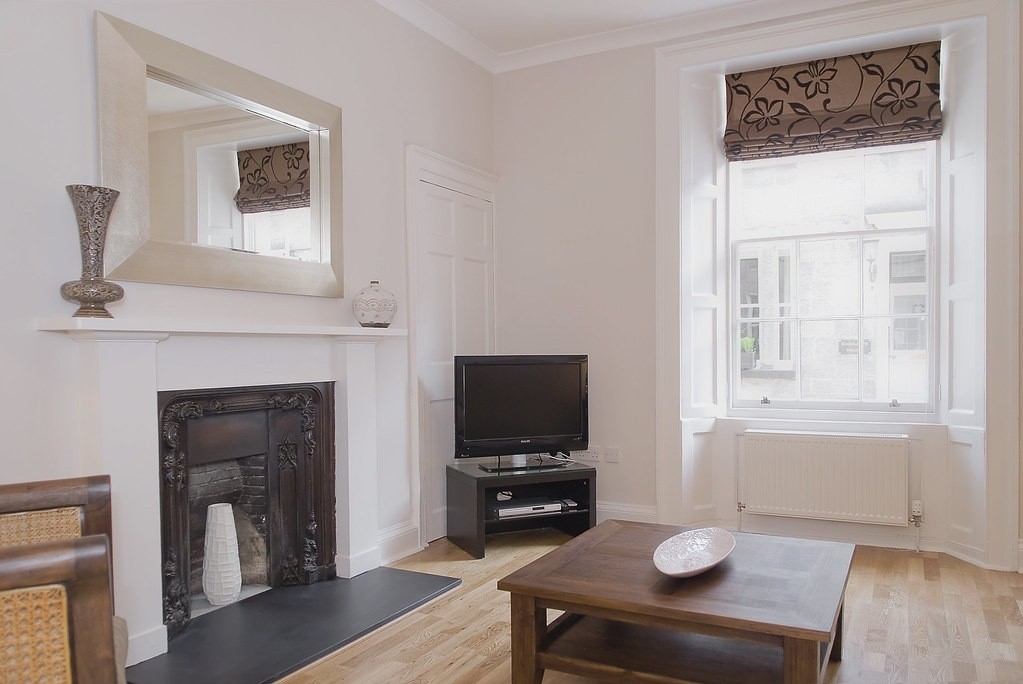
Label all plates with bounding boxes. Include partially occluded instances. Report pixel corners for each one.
[652,527,736,579]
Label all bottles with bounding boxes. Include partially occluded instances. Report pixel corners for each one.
[202,503,242,606]
[352,281,398,328]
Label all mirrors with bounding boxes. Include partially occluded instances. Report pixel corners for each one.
[94,10,344,299]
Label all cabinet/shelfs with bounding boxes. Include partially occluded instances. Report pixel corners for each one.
[446,457,596,560]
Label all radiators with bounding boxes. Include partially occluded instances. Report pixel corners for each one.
[742,429,911,528]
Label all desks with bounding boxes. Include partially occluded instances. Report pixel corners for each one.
[497,519,856,684]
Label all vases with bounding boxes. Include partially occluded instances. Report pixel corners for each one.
[60,184,124,318]
[201,503,243,606]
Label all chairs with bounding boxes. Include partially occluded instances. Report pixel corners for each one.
[1,474,129,684]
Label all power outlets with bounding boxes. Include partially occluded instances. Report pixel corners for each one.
[603,447,620,463]
[569,446,600,462]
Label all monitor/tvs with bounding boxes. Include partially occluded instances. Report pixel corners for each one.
[453,355,589,472]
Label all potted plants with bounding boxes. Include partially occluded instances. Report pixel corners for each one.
[740,336,757,372]
